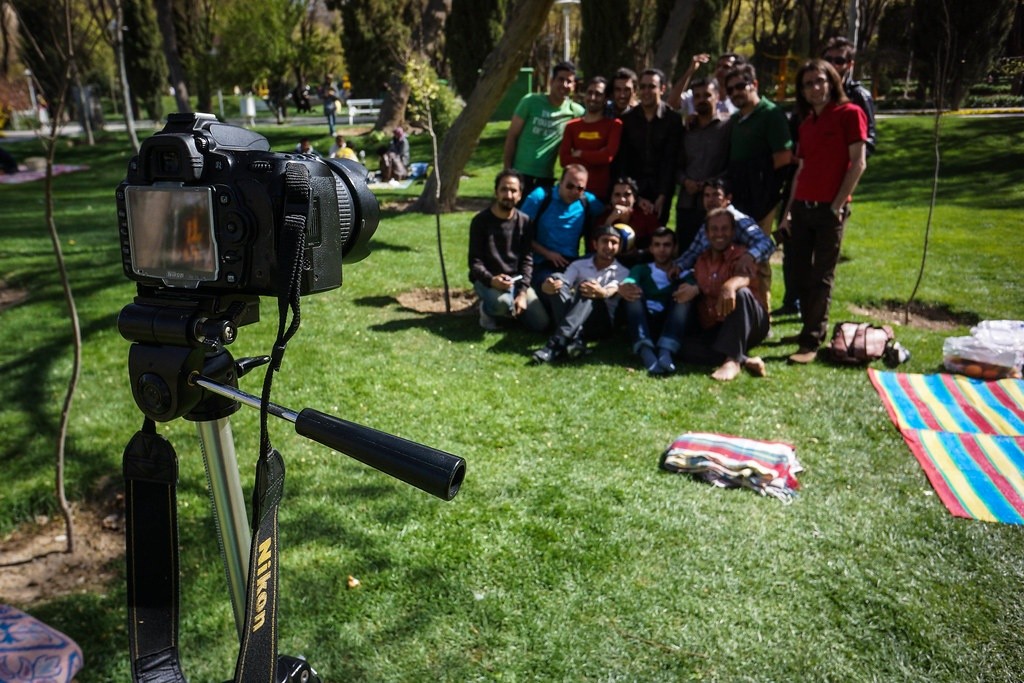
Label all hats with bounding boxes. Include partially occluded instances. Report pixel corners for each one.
[614,223,636,251]
[392,127,404,137]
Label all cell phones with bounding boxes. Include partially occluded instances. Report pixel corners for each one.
[508,275,523,282]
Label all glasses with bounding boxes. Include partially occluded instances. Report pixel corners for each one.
[726,82,750,96]
[612,191,633,197]
[806,78,827,88]
[561,181,585,192]
[825,55,849,65]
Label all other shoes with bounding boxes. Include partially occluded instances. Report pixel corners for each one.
[566,343,583,360]
[534,346,568,364]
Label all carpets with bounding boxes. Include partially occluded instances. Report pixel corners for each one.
[866,365,1024,526]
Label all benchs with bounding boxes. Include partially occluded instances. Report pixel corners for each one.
[347,98,384,126]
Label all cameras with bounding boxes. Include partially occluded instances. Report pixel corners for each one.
[116,112,381,297]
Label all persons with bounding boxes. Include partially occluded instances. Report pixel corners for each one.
[606,67,639,117]
[268,77,290,124]
[329,136,359,164]
[502,61,585,208]
[468,164,655,330]
[672,208,770,380]
[296,138,313,153]
[377,126,410,182]
[530,224,631,365]
[668,54,793,254]
[617,226,699,376]
[667,177,776,338]
[611,70,688,223]
[770,36,875,366]
[293,83,313,113]
[559,76,624,254]
[323,74,352,136]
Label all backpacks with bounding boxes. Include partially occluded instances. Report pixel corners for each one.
[833,320,894,357]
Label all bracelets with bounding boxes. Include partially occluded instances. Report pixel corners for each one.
[833,208,844,215]
